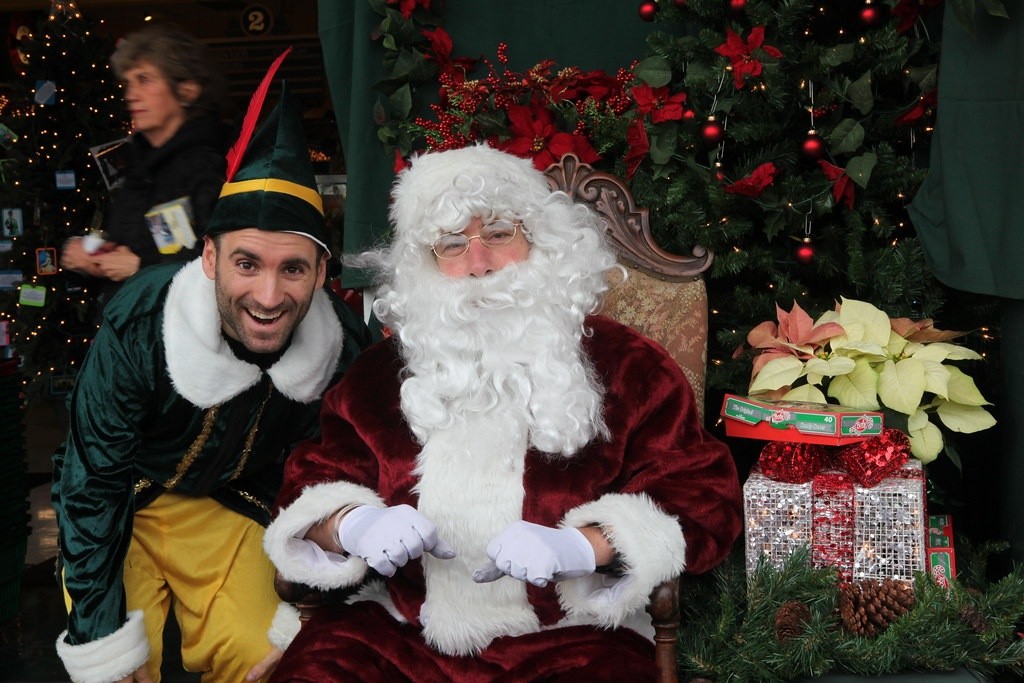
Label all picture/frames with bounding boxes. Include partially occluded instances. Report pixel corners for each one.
[2,208,23,237]
[50,374,76,394]
[36,247,57,275]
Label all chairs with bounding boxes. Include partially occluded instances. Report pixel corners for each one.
[274,153,714,683]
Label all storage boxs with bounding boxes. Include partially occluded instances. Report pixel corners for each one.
[925,514,957,597]
[721,393,883,447]
[745,458,925,620]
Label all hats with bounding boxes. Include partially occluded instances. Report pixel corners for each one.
[205,42,345,258]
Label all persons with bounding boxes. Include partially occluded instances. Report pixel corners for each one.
[41,253,55,272]
[56,82,375,683]
[61,28,239,319]
[266,145,742,683]
[5,211,19,235]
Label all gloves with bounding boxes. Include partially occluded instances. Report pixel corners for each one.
[469,519,597,588]
[340,503,458,582]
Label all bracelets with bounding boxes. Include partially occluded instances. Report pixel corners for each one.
[332,503,367,547]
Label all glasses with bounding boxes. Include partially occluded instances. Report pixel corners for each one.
[429,216,520,260]
